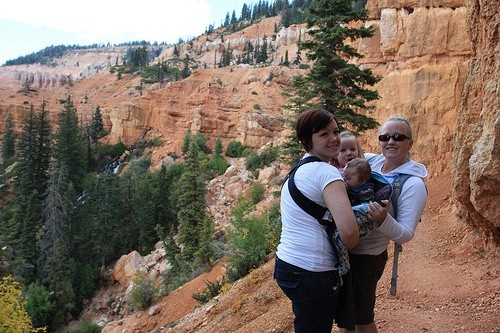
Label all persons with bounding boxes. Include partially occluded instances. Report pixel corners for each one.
[333,158,375,275]
[273,109,359,333]
[330,131,365,173]
[341,117,428,333]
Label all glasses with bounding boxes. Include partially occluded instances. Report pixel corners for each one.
[379,134,410,142]
